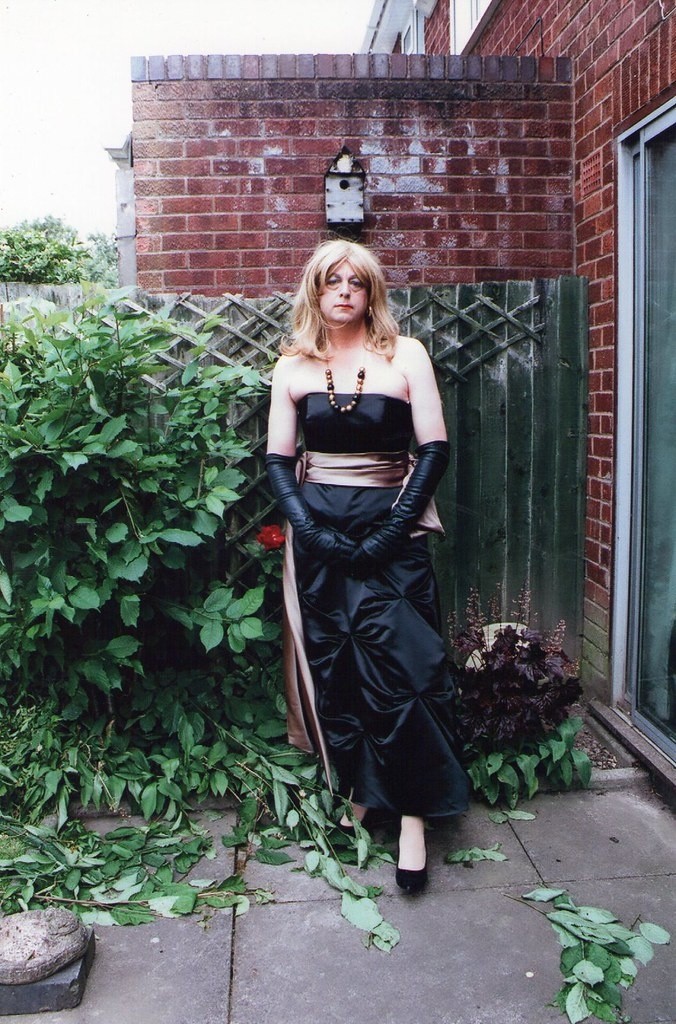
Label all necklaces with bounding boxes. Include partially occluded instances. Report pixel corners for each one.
[326,349,365,413]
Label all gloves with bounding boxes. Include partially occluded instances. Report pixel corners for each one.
[347,440,450,577]
[265,453,358,569]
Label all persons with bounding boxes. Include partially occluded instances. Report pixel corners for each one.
[266,240,474,892]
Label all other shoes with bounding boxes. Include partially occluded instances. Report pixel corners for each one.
[338,810,368,835]
[395,839,427,897]
